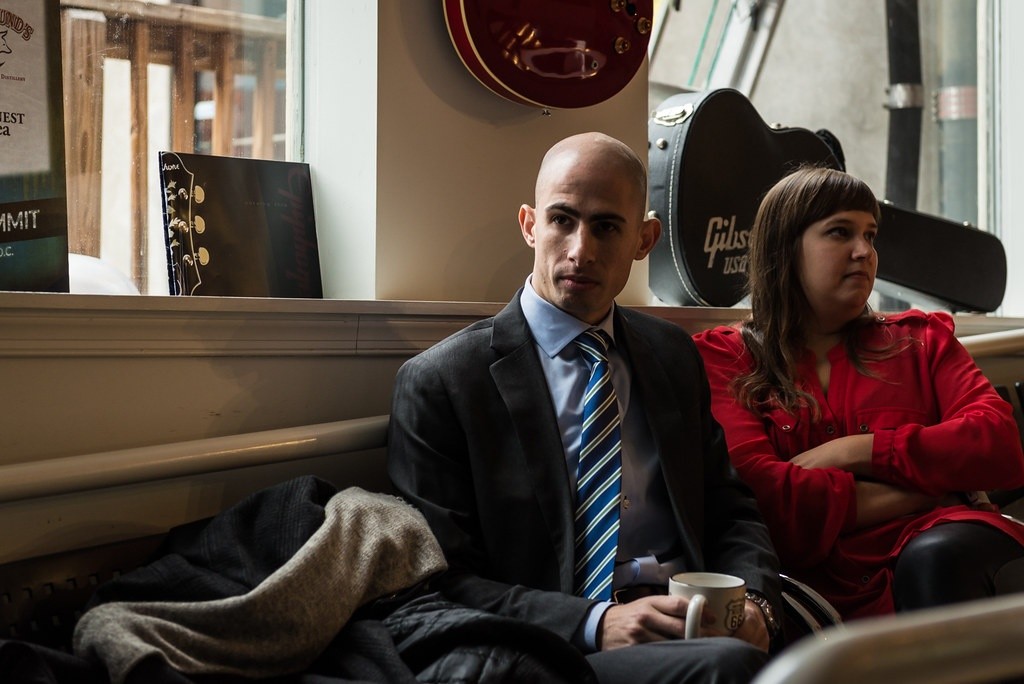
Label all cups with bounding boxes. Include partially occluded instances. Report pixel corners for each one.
[669,572,746,640]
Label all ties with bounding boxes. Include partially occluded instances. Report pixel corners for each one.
[571,326,623,604]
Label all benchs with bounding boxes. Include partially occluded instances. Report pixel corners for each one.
[0,330,1024,684]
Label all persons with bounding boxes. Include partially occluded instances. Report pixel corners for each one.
[385,134,788,683]
[692,164,1024,620]
